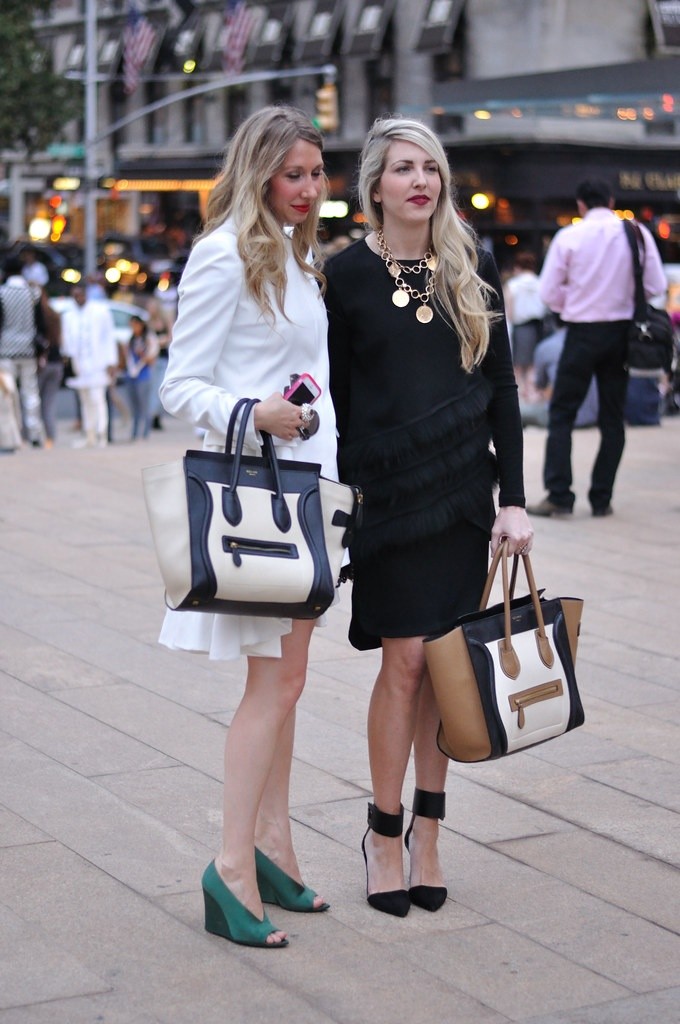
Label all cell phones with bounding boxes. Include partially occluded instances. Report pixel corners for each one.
[283,373,322,406]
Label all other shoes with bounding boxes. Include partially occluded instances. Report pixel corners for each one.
[525,500,572,515]
[592,505,613,516]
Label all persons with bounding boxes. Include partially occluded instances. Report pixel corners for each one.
[146,296,173,430]
[153,285,177,322]
[519,313,597,430]
[160,103,339,948]
[18,248,51,291]
[112,280,134,304]
[0,256,47,453]
[59,282,118,449]
[125,315,159,441]
[27,283,65,448]
[503,247,548,402]
[525,176,667,516]
[317,108,534,919]
[623,374,661,426]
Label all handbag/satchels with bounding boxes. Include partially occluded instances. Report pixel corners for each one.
[422,540,585,763]
[627,303,675,370]
[139,397,364,620]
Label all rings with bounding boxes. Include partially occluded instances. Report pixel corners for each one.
[520,545,528,553]
[301,403,314,422]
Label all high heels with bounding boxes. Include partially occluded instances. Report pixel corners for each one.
[202,859,289,947]
[404,788,447,912]
[254,844,331,913]
[361,802,411,918]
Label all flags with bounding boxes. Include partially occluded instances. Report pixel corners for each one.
[221,0,258,74]
[121,7,158,95]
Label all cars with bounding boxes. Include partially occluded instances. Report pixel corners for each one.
[0,232,192,343]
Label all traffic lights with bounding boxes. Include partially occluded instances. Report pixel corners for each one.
[310,84,340,135]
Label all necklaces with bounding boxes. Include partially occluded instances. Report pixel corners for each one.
[378,229,439,323]
